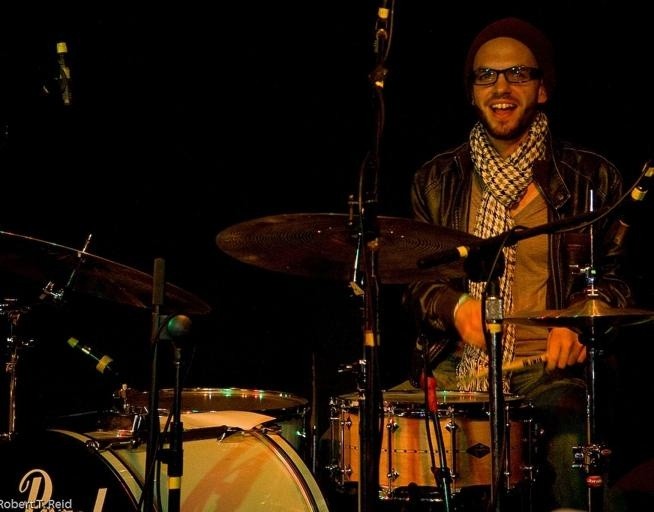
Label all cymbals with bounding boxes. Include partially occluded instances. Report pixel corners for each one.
[487,301,654,339]
[0,230,212,316]
[219,213,488,285]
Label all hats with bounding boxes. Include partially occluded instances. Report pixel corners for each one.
[465,17,553,73]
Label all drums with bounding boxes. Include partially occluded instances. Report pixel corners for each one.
[0,412,332,512]
[122,388,308,465]
[329,390,538,500]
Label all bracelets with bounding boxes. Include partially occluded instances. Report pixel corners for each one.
[451,288,472,321]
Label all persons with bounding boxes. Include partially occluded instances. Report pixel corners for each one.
[313,17,628,511]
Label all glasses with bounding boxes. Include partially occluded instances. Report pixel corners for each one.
[470,64,544,86]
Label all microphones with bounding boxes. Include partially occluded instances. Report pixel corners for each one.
[619,155,654,228]
[374,30,391,71]
[161,316,192,343]
[67,335,123,388]
[56,41,73,103]
